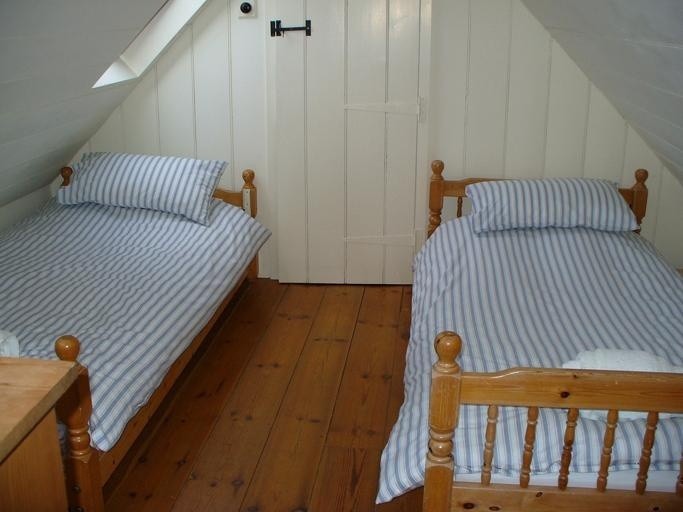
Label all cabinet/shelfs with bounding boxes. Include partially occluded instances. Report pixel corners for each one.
[0,353,79,510]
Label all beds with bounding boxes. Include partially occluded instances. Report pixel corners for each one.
[373,157,677,509]
[0,159,271,512]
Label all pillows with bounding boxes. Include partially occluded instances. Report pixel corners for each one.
[466,177,641,233]
[55,152,227,225]
